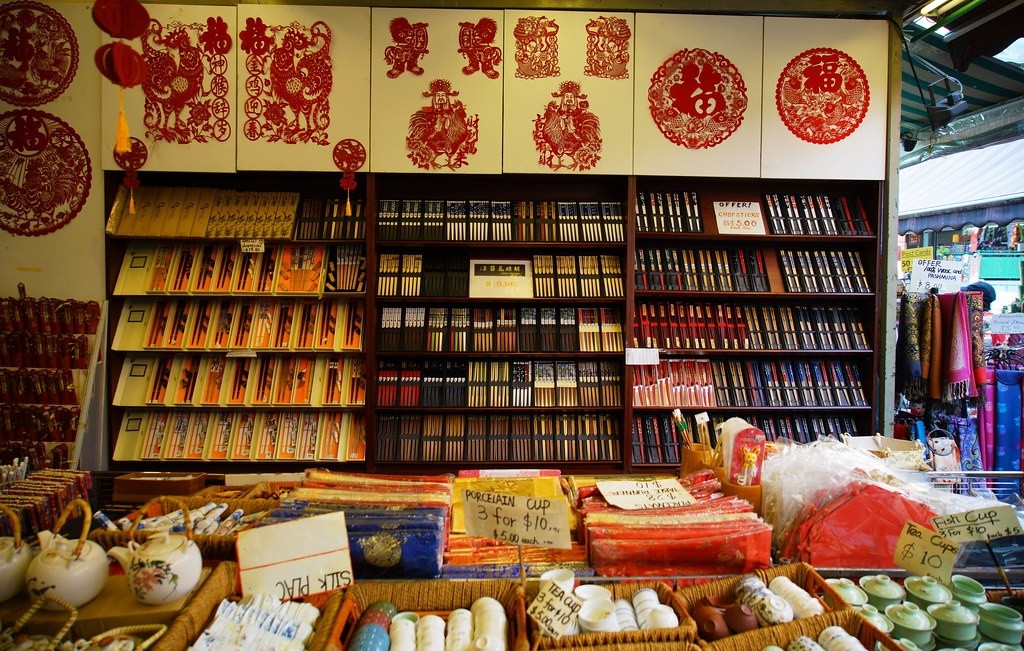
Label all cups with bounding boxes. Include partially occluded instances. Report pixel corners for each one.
[699,459,724,481]
[540,569,678,635]
[787,636,827,651]
[722,480,762,515]
[680,443,713,479]
[818,626,869,651]
[825,574,1024,651]
[762,645,784,651]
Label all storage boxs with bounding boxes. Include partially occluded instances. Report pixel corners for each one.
[111,471,207,504]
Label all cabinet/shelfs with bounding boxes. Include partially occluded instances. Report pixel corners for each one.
[0,301,102,472]
[99,0,904,476]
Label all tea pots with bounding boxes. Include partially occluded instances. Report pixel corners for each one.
[107,495,202,604]
[0,504,31,604]
[0,595,78,651]
[58,625,167,651]
[25,499,109,610]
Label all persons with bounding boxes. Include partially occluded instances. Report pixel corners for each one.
[960,282,996,311]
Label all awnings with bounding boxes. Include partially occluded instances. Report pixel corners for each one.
[898,138,1024,236]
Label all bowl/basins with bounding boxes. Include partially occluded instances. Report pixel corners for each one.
[347,597,509,651]
[691,575,824,639]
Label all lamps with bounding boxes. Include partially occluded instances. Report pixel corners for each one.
[902,40,969,131]
[900,127,920,153]
